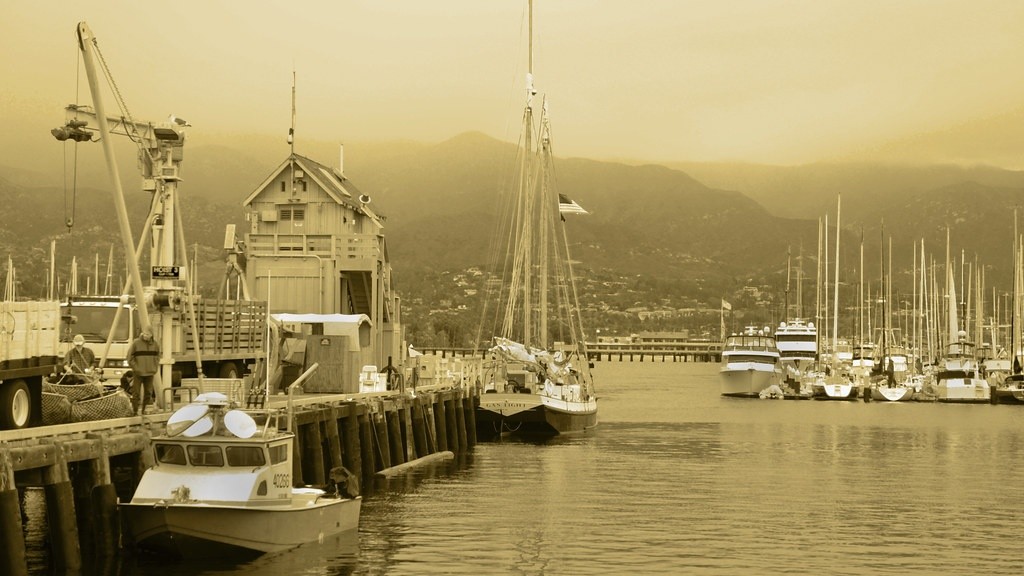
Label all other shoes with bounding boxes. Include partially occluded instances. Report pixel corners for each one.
[133,412,138,417]
[142,410,150,415]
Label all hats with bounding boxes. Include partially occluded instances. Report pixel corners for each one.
[73,334,85,346]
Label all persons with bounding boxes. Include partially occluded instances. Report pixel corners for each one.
[118,370,137,395]
[63,334,97,385]
[126,326,161,417]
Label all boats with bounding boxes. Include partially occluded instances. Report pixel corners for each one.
[116,391,363,563]
[718,320,783,396]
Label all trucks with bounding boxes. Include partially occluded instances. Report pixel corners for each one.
[59,295,269,406]
[0,300,60,428]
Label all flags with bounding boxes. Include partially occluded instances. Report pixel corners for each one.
[722,299,731,311]
[559,193,589,213]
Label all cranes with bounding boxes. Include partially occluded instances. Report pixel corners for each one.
[51,21,207,413]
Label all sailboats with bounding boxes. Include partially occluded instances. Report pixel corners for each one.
[472,0,602,438]
[776,191,1024,403]
